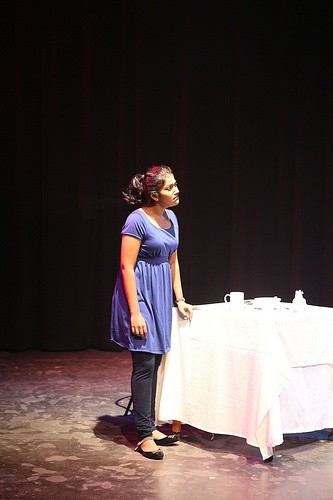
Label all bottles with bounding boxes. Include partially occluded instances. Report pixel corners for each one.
[292,290,307,312]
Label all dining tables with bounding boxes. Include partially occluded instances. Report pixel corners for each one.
[154,301,333,463]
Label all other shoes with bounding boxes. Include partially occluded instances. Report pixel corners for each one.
[154,434,178,445]
[137,443,163,459]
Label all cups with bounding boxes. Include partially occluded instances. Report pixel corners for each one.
[223,292,245,307]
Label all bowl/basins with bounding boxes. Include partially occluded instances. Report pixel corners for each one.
[253,297,281,308]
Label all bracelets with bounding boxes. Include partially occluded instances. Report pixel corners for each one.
[175,298,186,303]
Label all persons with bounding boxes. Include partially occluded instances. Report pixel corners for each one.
[110,165,193,459]
